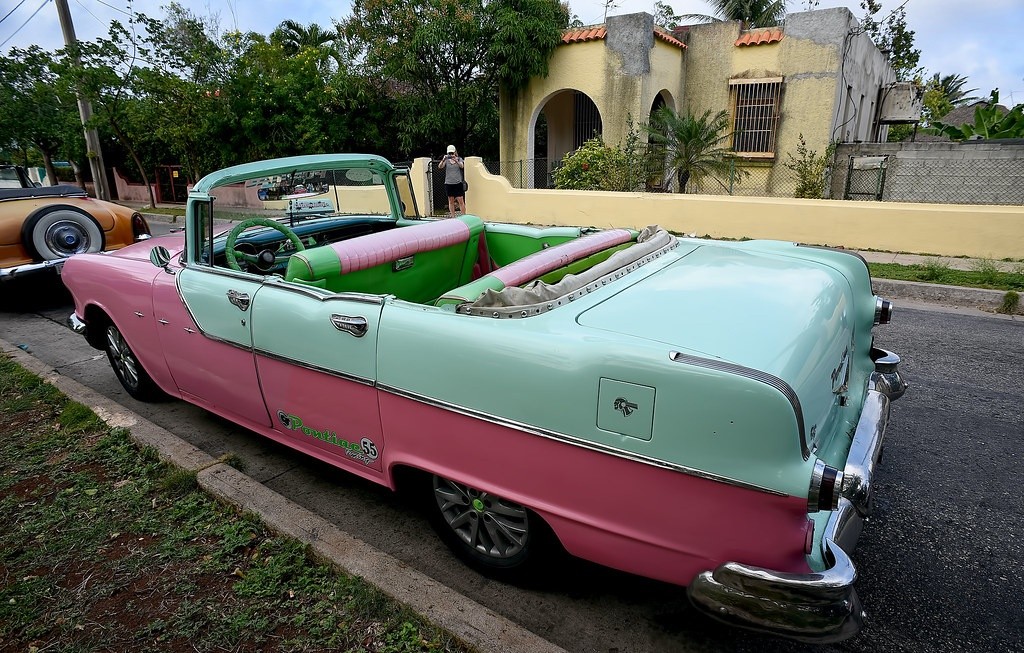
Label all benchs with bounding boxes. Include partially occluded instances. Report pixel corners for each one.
[435,227,641,308]
[286,214,484,305]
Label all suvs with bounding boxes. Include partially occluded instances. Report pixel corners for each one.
[0,164,153,282]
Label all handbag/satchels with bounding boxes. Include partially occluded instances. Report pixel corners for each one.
[460,180,468,193]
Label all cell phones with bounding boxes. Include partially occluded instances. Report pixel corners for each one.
[447,156,452,159]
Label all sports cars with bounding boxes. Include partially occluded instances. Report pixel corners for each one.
[59,153,906,643]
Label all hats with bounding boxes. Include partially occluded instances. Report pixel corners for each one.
[447,145,456,153]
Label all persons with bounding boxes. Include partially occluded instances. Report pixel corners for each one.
[438,145,466,218]
